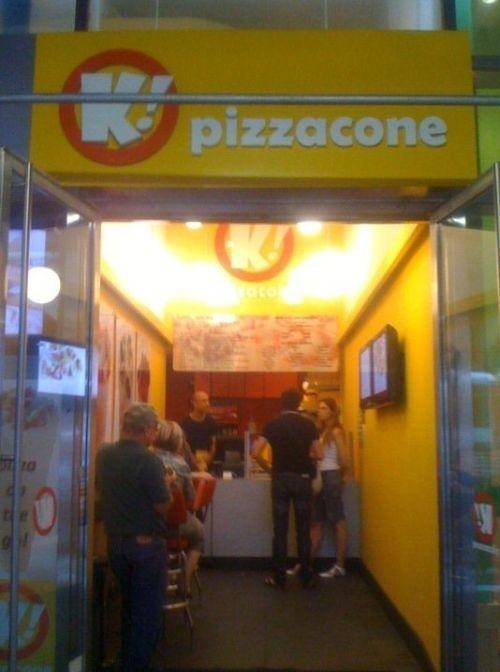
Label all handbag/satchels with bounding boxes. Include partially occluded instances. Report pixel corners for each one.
[311,474,323,496]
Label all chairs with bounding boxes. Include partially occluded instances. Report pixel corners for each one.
[161,476,218,652]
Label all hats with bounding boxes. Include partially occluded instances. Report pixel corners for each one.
[302,379,319,395]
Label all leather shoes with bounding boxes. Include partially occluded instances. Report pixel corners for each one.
[262,562,346,586]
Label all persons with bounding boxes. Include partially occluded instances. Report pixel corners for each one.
[250,386,325,588]
[91,400,179,671]
[149,417,209,606]
[283,395,355,580]
[176,390,218,472]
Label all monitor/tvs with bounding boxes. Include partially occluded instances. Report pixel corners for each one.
[26,334,89,399]
[360,325,400,407]
